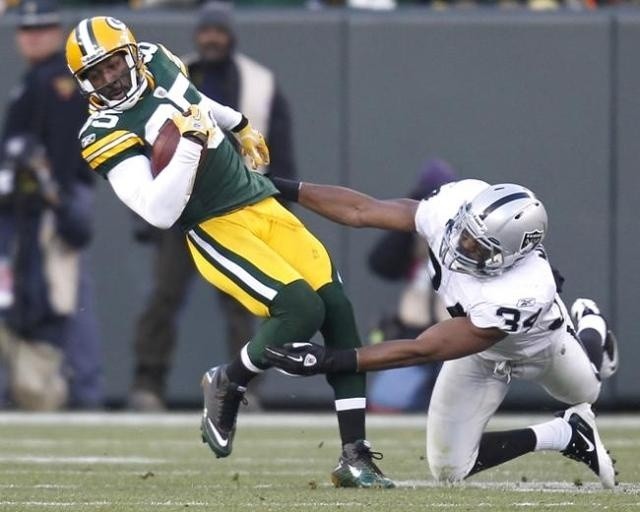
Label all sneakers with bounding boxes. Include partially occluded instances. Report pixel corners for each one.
[570,296,619,382]
[554,403,619,489]
[199,363,249,458]
[330,440,395,488]
[128,385,166,413]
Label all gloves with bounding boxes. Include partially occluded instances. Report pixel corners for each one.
[236,119,270,170]
[263,343,358,378]
[171,105,216,148]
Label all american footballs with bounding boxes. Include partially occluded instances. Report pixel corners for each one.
[150,111,192,179]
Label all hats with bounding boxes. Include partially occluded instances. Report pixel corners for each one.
[17,1,58,28]
[197,4,238,42]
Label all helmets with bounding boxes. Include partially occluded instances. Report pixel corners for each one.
[65,15,154,116]
[436,183,548,284]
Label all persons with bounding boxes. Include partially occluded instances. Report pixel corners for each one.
[260,171,621,492]
[0,1,114,411]
[126,1,303,418]
[62,14,395,494]
[364,155,461,413]
